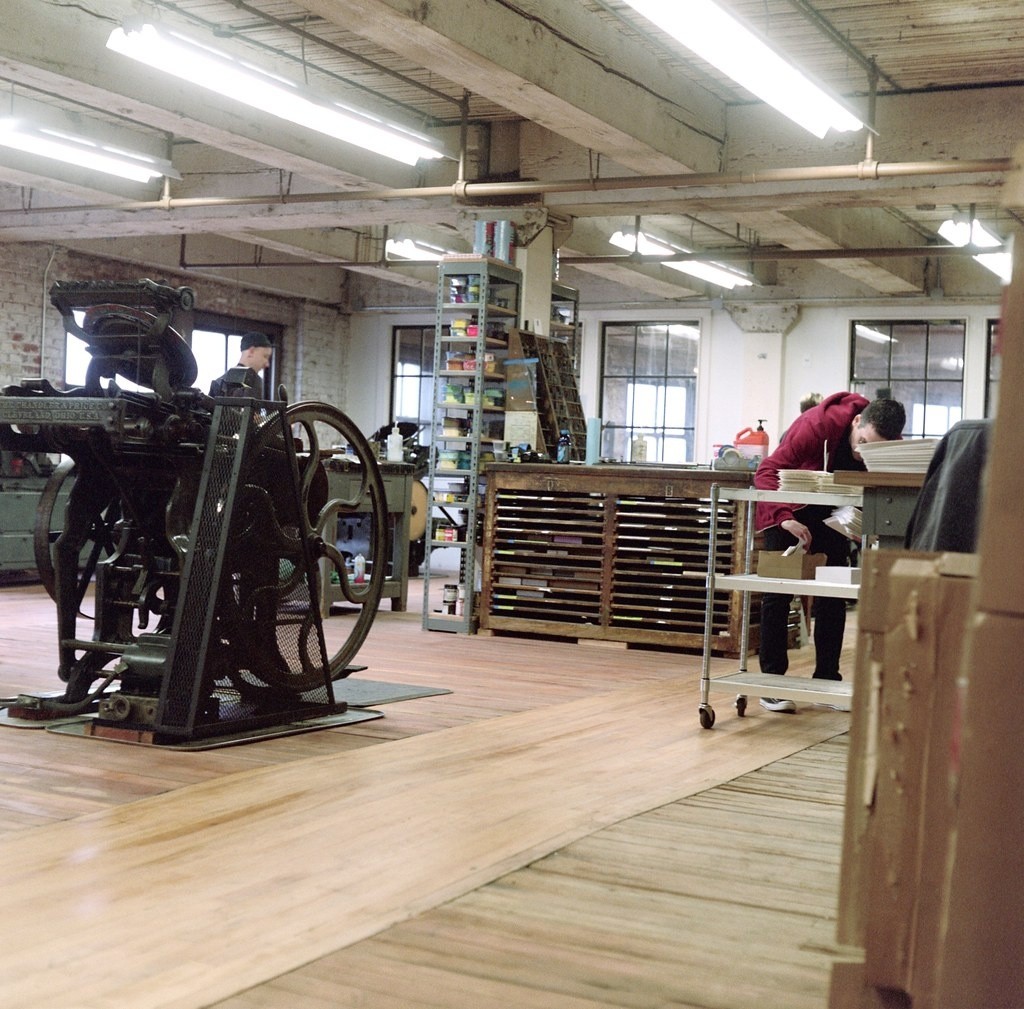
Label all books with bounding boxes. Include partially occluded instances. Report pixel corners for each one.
[822,505,863,543]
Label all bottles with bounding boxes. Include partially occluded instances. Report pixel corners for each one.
[441,583,466,617]
[557,429,572,463]
[354,554,365,583]
[733,419,769,472]
[387,427,404,462]
[713,444,723,458]
[632,433,647,463]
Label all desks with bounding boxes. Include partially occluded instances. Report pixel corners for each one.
[832,470,927,554]
[278,459,414,623]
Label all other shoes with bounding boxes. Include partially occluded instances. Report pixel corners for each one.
[814,702,851,711]
[759,697,795,711]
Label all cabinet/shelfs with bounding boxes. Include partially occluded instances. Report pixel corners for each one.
[695,481,861,715]
[423,253,522,638]
[478,460,803,661]
[550,279,580,367]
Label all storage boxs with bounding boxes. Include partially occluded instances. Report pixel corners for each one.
[815,566,861,584]
[757,550,826,579]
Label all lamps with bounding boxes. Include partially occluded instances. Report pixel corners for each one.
[604,212,757,289]
[0,81,184,184]
[384,221,467,270]
[619,0,881,152]
[937,216,1008,283]
[104,0,461,167]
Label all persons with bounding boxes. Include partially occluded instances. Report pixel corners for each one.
[753,392,906,712]
[208,331,273,417]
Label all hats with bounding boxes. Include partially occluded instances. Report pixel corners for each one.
[241,332,277,351]
